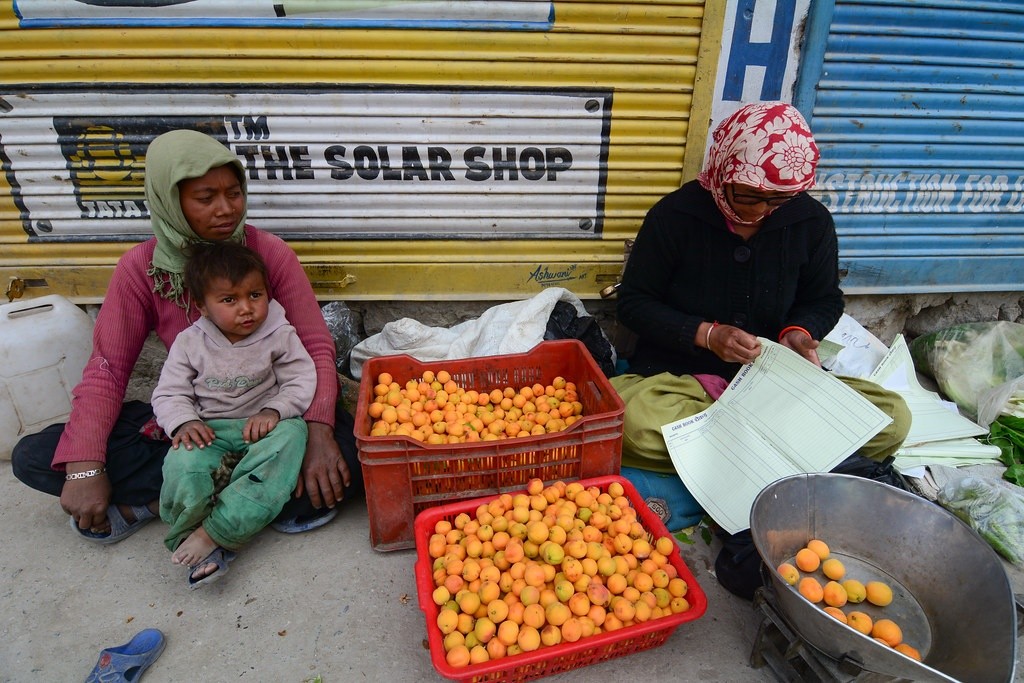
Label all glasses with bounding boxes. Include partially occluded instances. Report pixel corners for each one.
[730,183,799,207]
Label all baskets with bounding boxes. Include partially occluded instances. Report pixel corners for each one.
[410,474,709,683]
[353,336,628,553]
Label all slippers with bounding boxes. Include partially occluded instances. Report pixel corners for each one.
[68,496,162,544]
[185,545,238,591]
[86,628,166,683]
[265,497,342,534]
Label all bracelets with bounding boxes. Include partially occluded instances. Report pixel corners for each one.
[778,325,813,343]
[706,322,718,351]
[65,466,107,482]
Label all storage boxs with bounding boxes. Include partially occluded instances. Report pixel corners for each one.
[414,474,708,683]
[353,340,626,554]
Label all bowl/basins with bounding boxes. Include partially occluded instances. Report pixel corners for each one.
[749,470,1018,683]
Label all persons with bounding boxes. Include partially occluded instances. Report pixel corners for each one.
[10,126,352,536]
[602,98,915,475]
[148,235,318,594]
